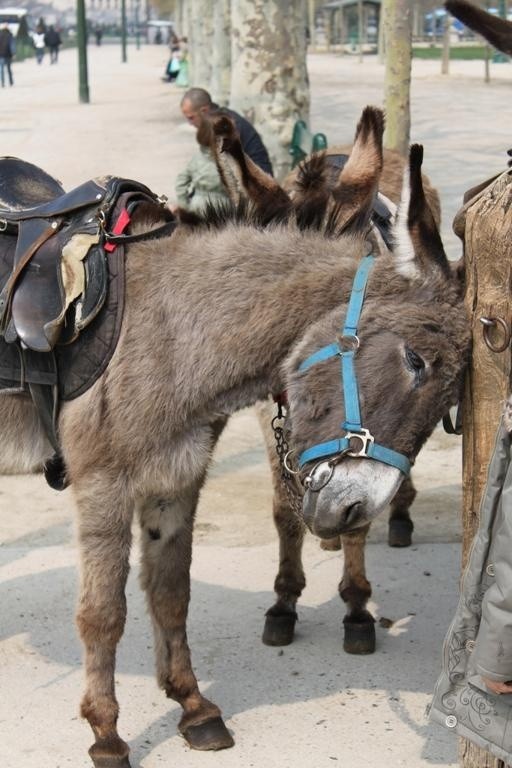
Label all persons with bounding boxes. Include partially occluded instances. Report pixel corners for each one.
[0,20,16,87]
[181,88,274,178]
[44,25,62,65]
[161,26,187,82]
[176,110,240,218]
[30,24,48,65]
[94,26,103,46]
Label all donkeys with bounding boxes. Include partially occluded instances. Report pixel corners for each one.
[0,143,475,768]
[209,107,441,655]
